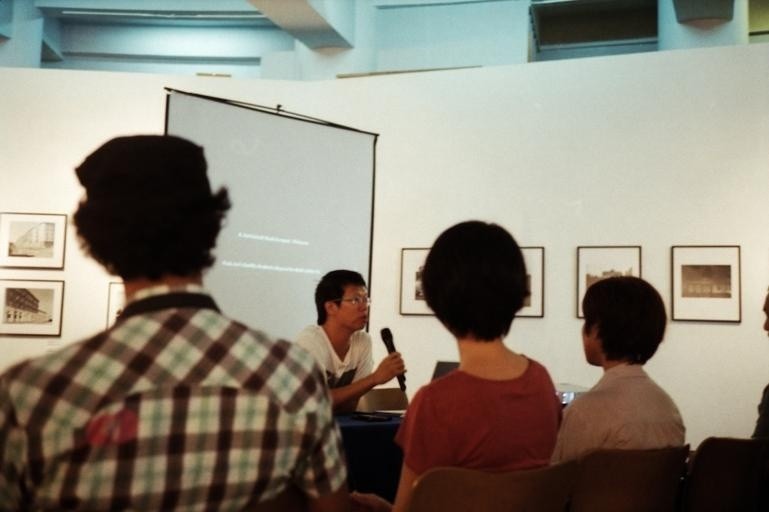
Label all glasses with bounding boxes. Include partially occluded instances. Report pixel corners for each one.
[333,295,370,305]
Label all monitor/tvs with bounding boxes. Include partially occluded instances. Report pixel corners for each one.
[431,361,460,382]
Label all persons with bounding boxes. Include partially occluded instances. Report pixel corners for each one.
[282,270,405,417]
[745,288,769,444]
[350,221,561,512]
[0,131,350,512]
[550,274,687,462]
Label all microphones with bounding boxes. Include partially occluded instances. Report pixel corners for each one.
[380,328,410,391]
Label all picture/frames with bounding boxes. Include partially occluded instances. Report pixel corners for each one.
[669,245,743,326]
[576,246,643,320]
[398,246,544,318]
[0,208,67,339]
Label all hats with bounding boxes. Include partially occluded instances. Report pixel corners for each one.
[75,135,215,199]
